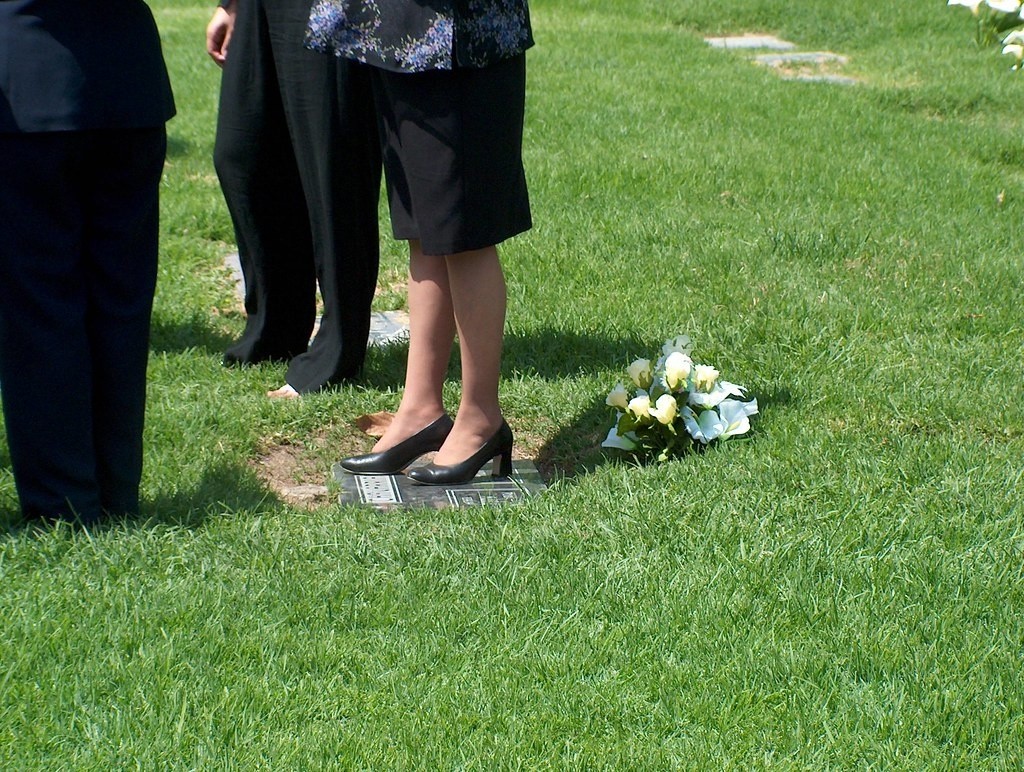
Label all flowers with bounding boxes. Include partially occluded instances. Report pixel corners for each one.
[602,336,758,462]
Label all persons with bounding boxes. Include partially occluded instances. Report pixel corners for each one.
[0,1,179,527]
[302,1,538,485]
[203,0,385,399]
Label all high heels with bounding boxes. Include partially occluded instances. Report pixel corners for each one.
[407,415,513,486]
[340,413,456,475]
